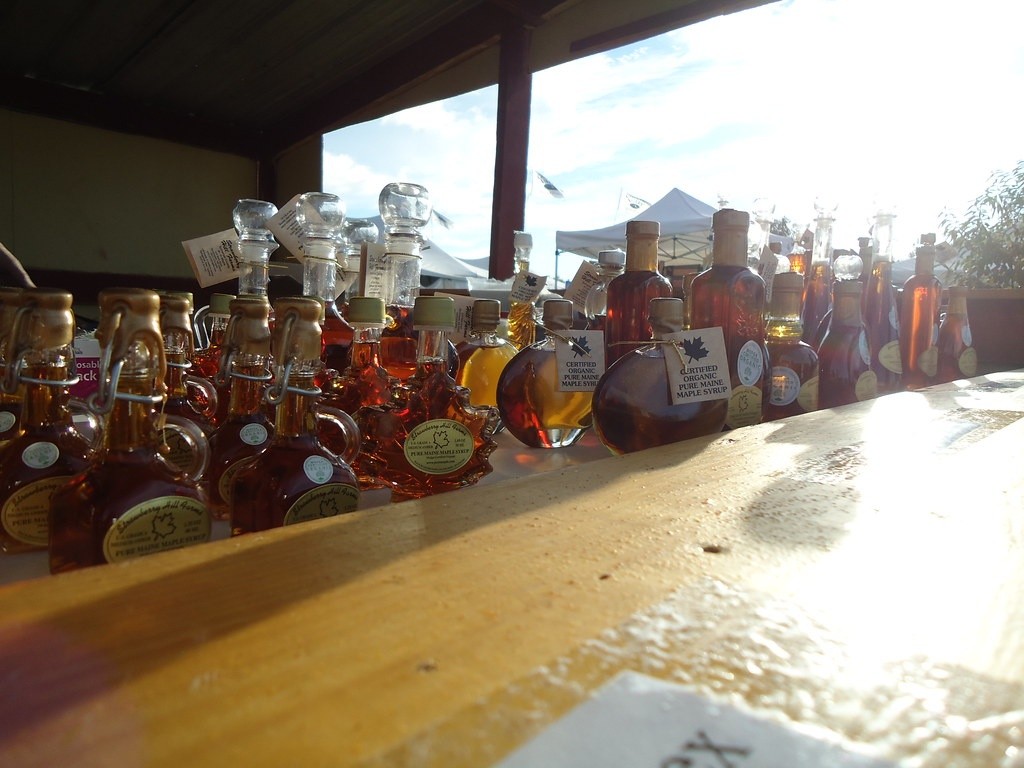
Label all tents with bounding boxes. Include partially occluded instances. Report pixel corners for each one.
[554,187,797,293]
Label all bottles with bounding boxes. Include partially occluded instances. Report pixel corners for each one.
[0,182,977,576]
